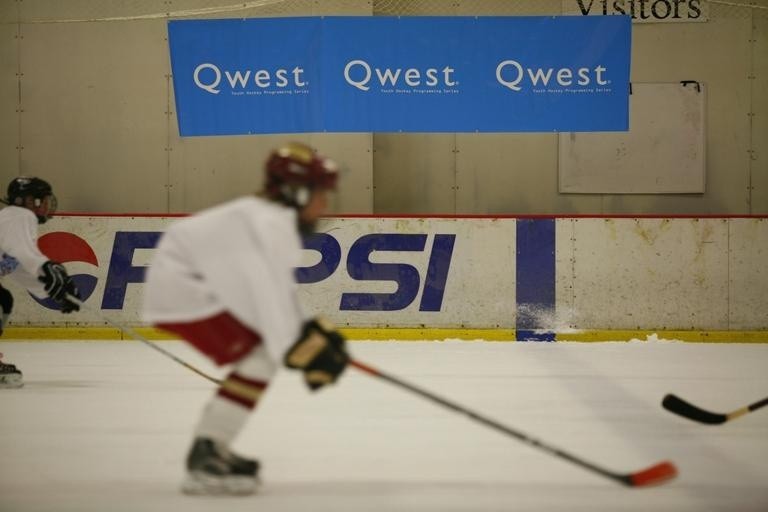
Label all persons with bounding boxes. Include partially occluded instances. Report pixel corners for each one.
[0,176,82,380]
[140,142,349,477]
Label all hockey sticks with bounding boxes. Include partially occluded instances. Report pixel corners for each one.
[344,353,678,488]
[661,391,767,426]
[66,294,225,387]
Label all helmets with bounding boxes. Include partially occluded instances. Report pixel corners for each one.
[261,140,342,211]
[8,176,57,223]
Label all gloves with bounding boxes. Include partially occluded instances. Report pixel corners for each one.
[283,320,350,389]
[39,261,82,314]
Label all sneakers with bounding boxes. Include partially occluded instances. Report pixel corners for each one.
[187,439,259,474]
[0,357,18,375]
